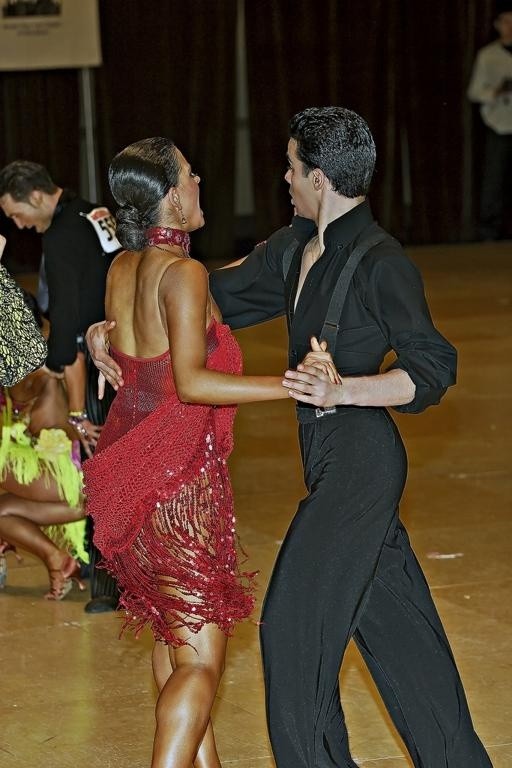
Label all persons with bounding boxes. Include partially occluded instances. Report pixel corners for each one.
[1,160,133,616]
[467,12,512,240]
[76,132,343,766]
[81,104,494,767]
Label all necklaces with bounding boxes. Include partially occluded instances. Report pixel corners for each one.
[146,225,191,249]
[155,246,186,259]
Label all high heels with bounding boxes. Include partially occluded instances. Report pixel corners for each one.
[0,542,24,564]
[44,557,85,600]
